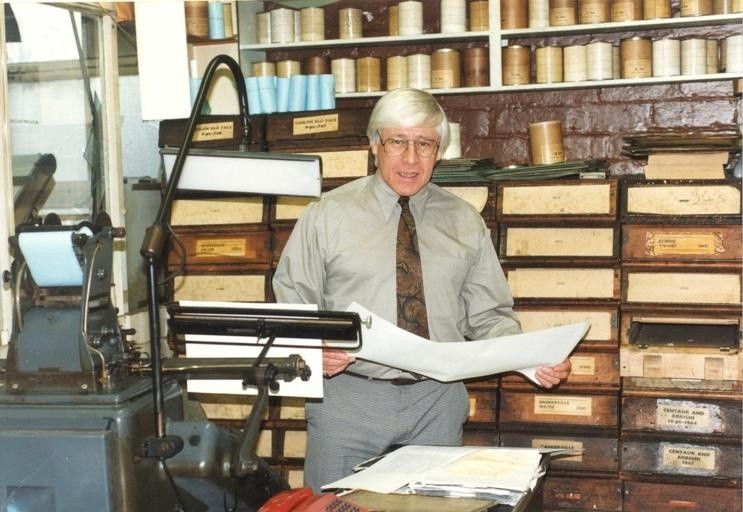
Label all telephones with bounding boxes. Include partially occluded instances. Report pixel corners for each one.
[257,487,369,512]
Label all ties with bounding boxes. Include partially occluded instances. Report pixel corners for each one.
[397,196,430,379]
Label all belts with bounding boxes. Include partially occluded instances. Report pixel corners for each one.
[341,370,429,384]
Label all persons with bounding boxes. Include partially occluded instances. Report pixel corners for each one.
[269,86,573,491]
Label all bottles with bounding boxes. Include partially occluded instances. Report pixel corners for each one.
[185,1,238,44]
[240,4,491,98]
[499,1,741,83]
[529,120,566,167]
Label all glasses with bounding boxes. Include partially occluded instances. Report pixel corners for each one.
[376,129,440,157]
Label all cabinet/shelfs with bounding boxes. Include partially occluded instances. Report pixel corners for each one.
[234,0,743,101]
